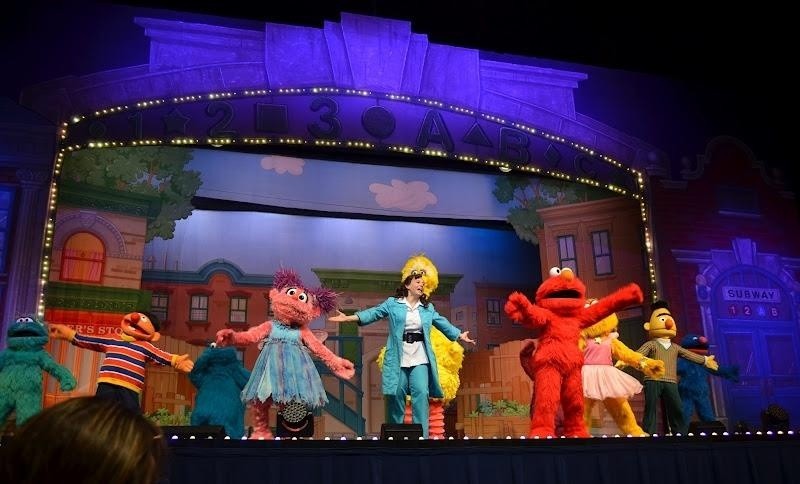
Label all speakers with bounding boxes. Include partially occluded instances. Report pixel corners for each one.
[160,425,226,440]
[687,420,727,435]
[380,424,423,440]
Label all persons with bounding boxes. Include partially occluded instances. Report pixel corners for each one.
[1,395,167,484]
[327,274,477,439]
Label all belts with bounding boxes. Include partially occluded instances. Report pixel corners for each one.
[402,332,424,342]
